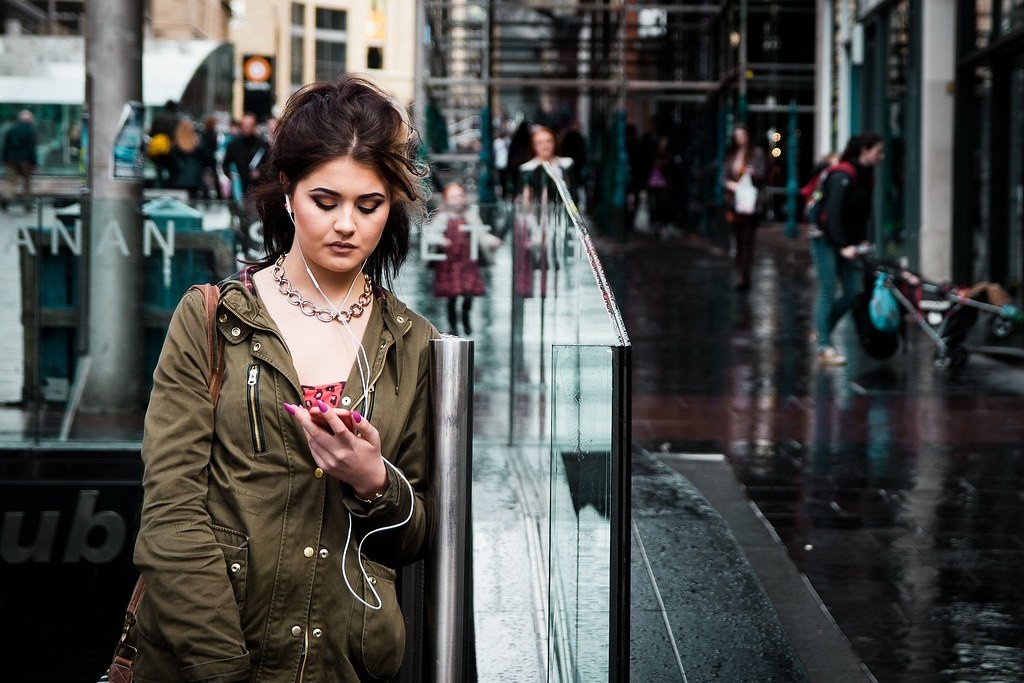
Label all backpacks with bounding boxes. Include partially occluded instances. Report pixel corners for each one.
[801,163,876,223]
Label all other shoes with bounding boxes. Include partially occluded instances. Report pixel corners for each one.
[814,346,847,365]
[736,275,750,295]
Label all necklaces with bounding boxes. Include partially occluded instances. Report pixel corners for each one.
[272,255,374,324]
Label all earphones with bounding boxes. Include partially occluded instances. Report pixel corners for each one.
[285,194,292,214]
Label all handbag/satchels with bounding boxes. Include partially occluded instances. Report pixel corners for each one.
[734,174,758,214]
[635,191,652,235]
[854,272,903,360]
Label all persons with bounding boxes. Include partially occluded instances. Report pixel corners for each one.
[713,125,771,273]
[106,76,440,681]
[799,132,885,365]
[0,99,683,336]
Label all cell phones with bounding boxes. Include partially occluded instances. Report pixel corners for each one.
[309,406,356,436]
